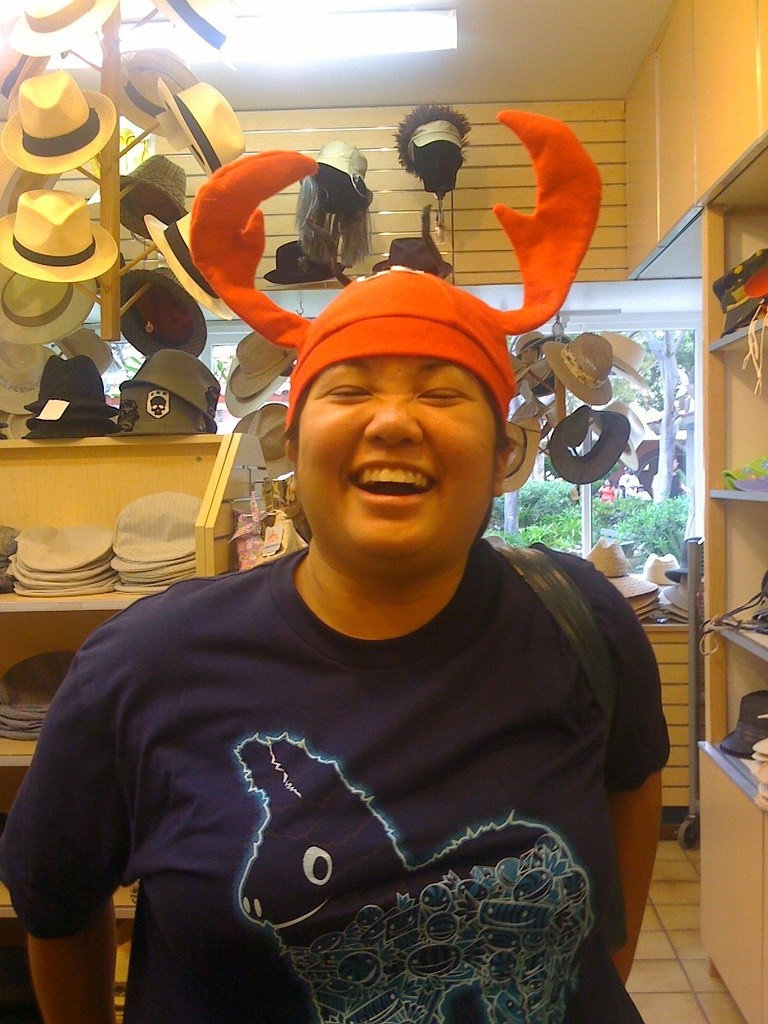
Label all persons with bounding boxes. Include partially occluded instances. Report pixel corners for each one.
[0,269,670,1024]
[598,458,686,505]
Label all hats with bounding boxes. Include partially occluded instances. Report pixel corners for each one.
[0,0,768,815]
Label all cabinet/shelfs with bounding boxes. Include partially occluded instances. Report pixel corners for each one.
[625,0,768,1023]
[0,428,273,1024]
